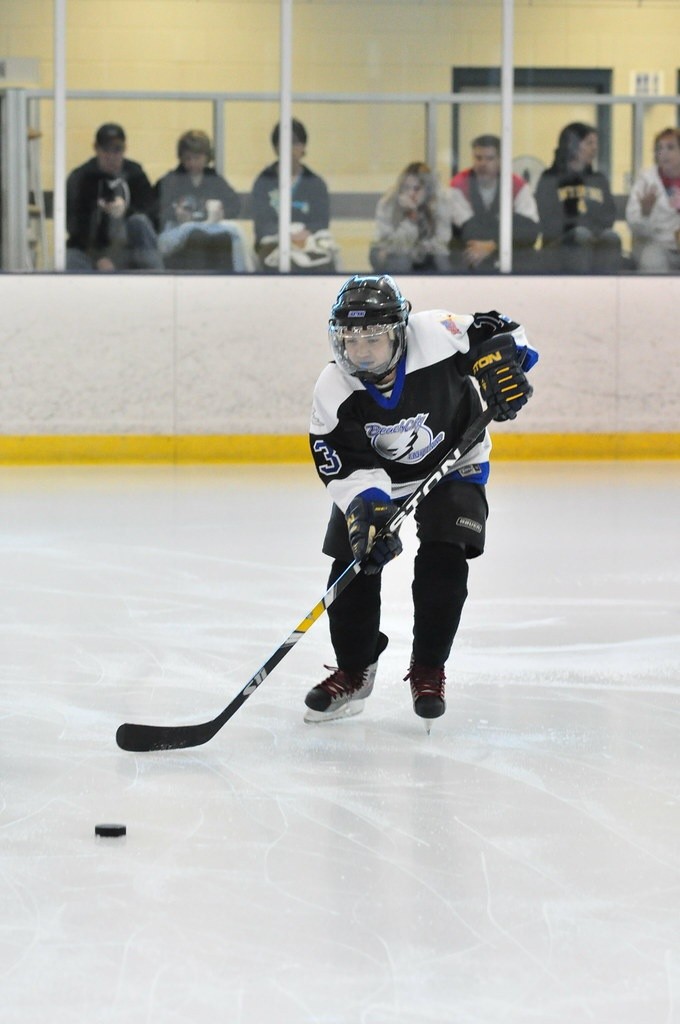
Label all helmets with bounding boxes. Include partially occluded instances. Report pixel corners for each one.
[328,274,409,328]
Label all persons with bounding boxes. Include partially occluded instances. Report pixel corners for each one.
[369,122,680,274]
[66,119,335,273]
[301,275,533,736]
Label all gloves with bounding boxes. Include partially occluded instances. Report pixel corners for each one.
[344,495,404,575]
[468,334,533,422]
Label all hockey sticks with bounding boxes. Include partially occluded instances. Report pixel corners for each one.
[116,406,496,752]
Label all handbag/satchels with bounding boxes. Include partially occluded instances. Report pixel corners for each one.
[264,228,336,268]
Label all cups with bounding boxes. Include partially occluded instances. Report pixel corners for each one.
[289,222,304,249]
[205,199,221,222]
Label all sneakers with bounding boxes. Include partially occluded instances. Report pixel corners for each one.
[404,654,446,735]
[303,659,379,722]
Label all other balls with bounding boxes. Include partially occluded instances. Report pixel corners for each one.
[94,823,126,838]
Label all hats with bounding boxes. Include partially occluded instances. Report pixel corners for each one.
[96,124,124,147]
